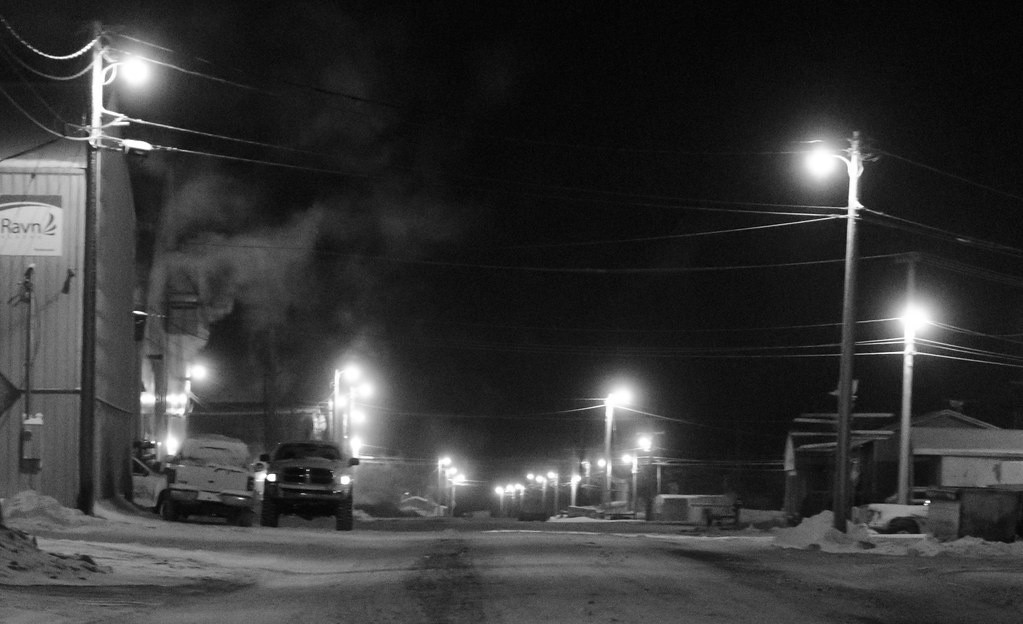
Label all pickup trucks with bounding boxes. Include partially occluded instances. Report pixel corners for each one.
[165,438,263,526]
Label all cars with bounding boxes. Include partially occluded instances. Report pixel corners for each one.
[131,454,168,517]
[260,438,362,531]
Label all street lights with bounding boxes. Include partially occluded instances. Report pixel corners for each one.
[497,453,638,514]
[333,366,370,444]
[75,59,151,517]
[603,390,630,457]
[898,305,929,504]
[438,457,466,516]
[809,151,861,535]
